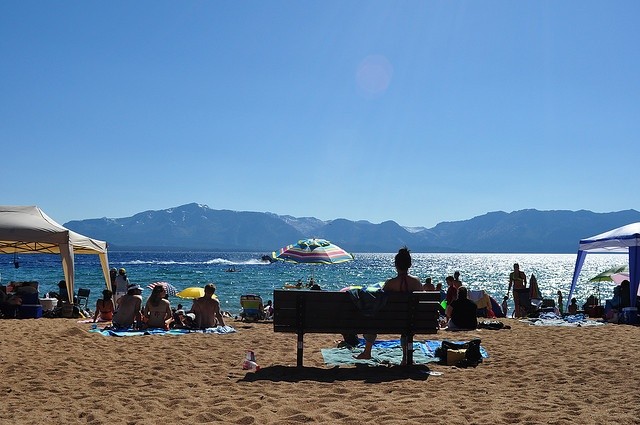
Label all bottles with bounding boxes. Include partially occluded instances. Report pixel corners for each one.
[242,360,261,372]
[133,321,138,330]
[46,293,49,298]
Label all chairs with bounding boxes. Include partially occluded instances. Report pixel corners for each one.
[239,295,268,322]
[73,288,90,310]
[512,288,555,319]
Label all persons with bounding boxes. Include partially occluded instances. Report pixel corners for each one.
[436,283,447,318]
[446,276,457,306]
[306,279,310,287]
[223,311,229,317]
[93,290,115,323]
[568,297,577,314]
[309,278,315,287]
[310,284,321,290]
[502,296,509,317]
[557,290,563,315]
[296,279,302,287]
[109,267,118,303]
[423,278,435,291]
[114,268,130,310]
[178,303,183,310]
[112,283,145,329]
[192,283,225,330]
[50,280,80,307]
[602,279,630,320]
[454,270,462,289]
[506,263,527,319]
[264,300,273,318]
[221,310,224,316]
[446,286,478,330]
[141,284,172,330]
[353,245,423,365]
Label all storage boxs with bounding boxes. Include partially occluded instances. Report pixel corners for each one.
[18,304,42,319]
[39,297,58,314]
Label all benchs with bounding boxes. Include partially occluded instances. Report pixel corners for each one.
[273,290,441,372]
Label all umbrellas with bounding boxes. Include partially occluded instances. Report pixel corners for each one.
[272,239,354,278]
[177,287,218,300]
[146,281,180,299]
[529,273,543,298]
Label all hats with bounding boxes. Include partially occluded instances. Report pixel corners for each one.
[57,281,66,285]
[119,267,126,273]
[110,266,117,271]
[128,284,145,290]
